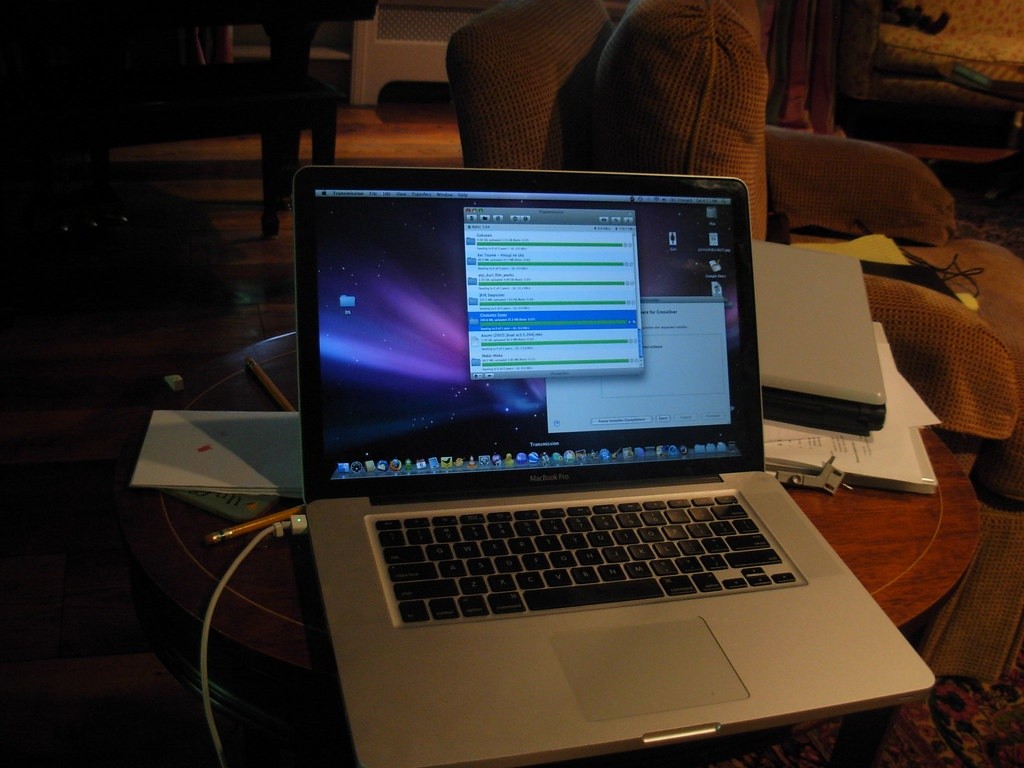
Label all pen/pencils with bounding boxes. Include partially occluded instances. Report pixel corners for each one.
[204,504,305,553]
[243,353,296,412]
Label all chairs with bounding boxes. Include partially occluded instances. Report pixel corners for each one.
[0,0,378,238]
[445,0,1024,441]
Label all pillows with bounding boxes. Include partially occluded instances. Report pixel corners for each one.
[583,1,769,243]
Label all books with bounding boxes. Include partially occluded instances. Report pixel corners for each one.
[953,61,1024,89]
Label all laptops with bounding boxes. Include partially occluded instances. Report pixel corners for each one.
[293,157,934,766]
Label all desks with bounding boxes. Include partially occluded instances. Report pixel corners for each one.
[104,329,982,768]
